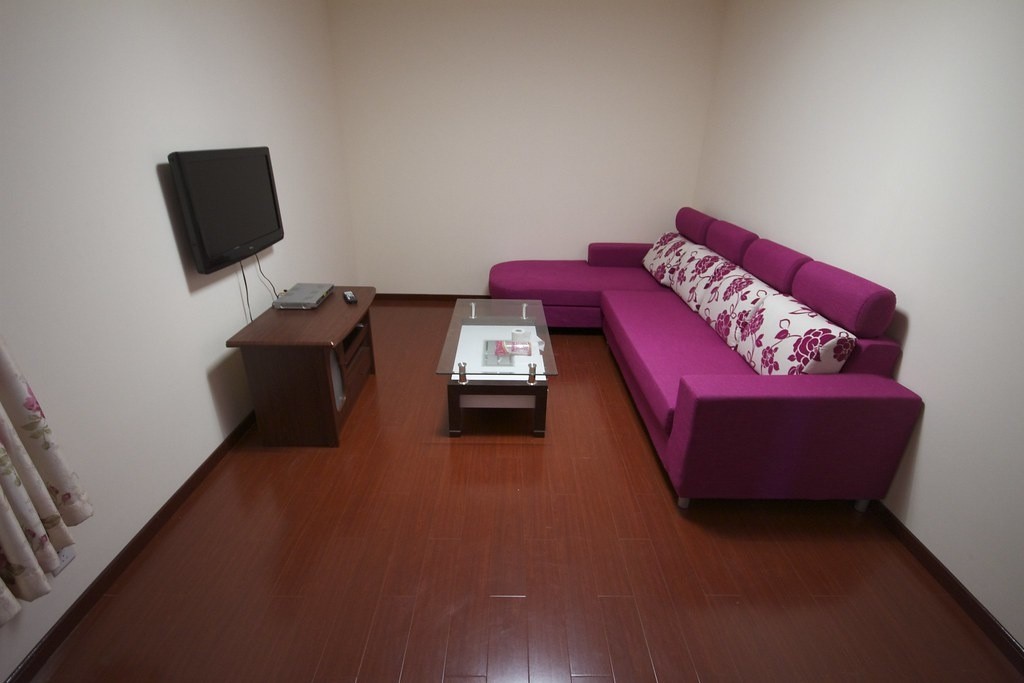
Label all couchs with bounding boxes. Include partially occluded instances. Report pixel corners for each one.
[491,205,926,523]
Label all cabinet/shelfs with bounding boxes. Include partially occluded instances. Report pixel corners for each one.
[226,285,380,450]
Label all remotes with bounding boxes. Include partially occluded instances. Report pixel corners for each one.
[343,290,357,304]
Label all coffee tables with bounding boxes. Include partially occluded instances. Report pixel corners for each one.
[436,299,559,437]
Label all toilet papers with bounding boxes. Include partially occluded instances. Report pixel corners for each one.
[512,328,545,351]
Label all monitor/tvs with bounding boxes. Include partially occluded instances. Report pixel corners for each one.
[168,146,285,274]
